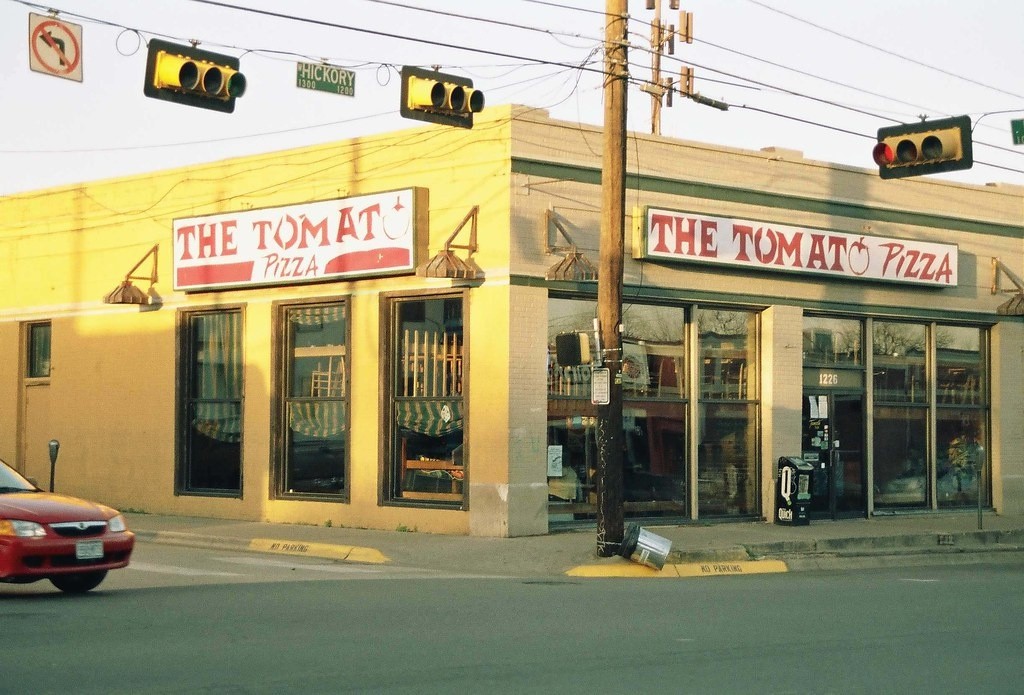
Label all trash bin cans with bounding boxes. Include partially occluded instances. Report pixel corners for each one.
[774,456,815,526]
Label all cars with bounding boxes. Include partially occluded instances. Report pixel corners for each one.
[0,458,137,593]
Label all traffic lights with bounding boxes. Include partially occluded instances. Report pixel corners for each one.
[872,115,973,179]
[555,331,590,367]
[143,37,247,114]
[399,66,485,129]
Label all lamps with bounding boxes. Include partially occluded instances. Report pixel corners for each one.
[416,206,478,280]
[991,257,1024,315]
[102,245,158,303]
[544,209,599,283]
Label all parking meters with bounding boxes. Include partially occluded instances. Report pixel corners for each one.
[48,439,60,493]
[973,444,986,529]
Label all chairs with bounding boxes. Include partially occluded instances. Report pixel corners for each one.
[311,356,346,397]
